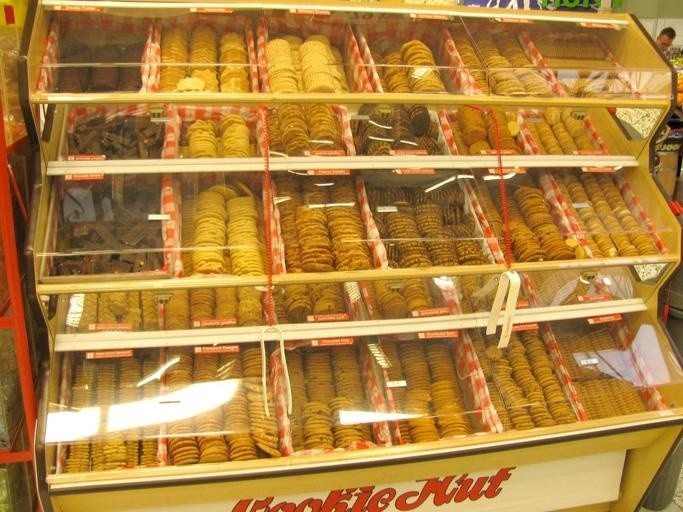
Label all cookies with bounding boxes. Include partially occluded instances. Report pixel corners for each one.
[48,23,661,474]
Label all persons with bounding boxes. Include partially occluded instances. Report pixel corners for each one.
[656,27,676,52]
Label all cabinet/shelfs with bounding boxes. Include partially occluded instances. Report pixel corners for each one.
[18,0,683,512]
[0,90,42,512]
